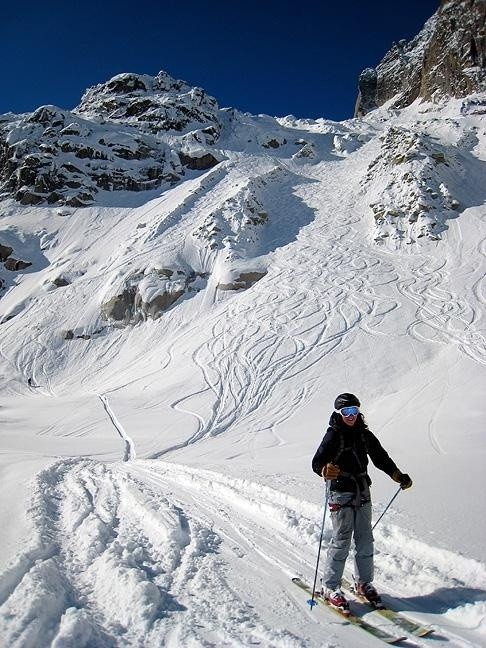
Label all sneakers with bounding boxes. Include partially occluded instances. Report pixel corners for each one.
[321,582,351,615]
[354,578,385,609]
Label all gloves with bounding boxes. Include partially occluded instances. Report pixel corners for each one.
[320,464,340,480]
[392,469,413,490]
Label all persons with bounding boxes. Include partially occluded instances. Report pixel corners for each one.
[311,392,414,616]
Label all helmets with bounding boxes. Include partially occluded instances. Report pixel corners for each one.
[334,392,360,409]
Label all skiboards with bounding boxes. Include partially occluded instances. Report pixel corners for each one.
[292,577,435,644]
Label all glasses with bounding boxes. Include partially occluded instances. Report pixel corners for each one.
[334,406,361,418]
